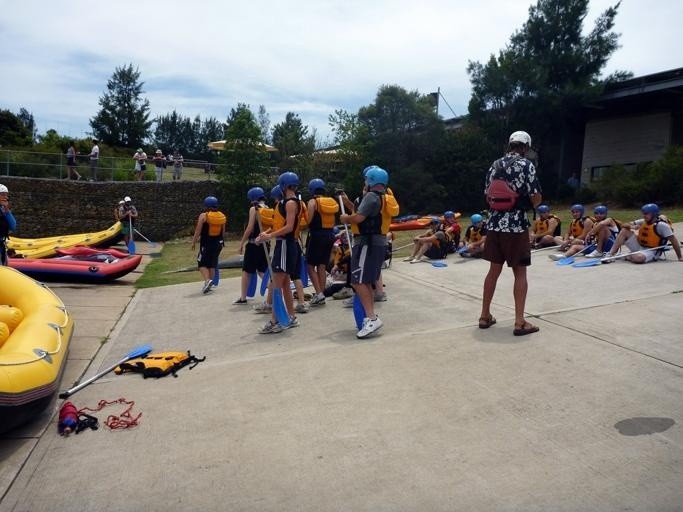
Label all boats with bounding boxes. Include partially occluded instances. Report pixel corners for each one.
[4,217,128,258]
[0,263,72,406]
[1,248,143,285]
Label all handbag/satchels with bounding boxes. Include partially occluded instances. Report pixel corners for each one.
[516,184,531,211]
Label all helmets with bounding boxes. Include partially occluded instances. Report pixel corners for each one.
[137,148,143,153]
[431,218,440,223]
[278,172,299,190]
[537,205,549,213]
[642,204,659,216]
[508,130,532,148]
[470,214,483,222]
[271,185,282,200]
[247,187,264,200]
[444,211,453,217]
[365,167,389,188]
[594,205,608,213]
[362,165,374,176]
[0,185,8,193]
[571,204,584,214]
[203,196,219,207]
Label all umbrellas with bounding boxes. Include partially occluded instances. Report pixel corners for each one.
[313,147,361,170]
[287,151,327,163]
[207,138,278,154]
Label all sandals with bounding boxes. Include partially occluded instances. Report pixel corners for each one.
[513,321,539,335]
[478,314,496,329]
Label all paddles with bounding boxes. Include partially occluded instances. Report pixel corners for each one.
[134,228,156,248]
[339,194,366,331]
[299,194,308,288]
[214,264,219,286]
[556,242,596,265]
[572,245,668,266]
[247,270,257,297]
[59,345,151,398]
[260,267,270,296]
[421,259,447,267]
[251,200,289,326]
[129,215,135,254]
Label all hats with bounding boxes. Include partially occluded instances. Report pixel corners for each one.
[155,149,162,154]
[309,179,326,192]
[125,196,131,202]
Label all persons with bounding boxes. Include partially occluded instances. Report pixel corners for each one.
[231,187,274,305]
[477,129,543,336]
[440,211,460,253]
[131,148,147,181]
[528,205,561,249]
[300,178,341,306]
[87,139,99,182]
[0,183,16,266]
[63,140,81,181]
[253,184,310,313]
[190,196,226,294]
[458,213,487,258]
[152,149,166,182]
[118,195,139,246]
[338,168,390,338]
[342,164,400,308]
[119,200,125,207]
[403,218,447,264]
[255,171,303,334]
[6,248,26,259]
[114,203,121,223]
[547,205,621,261]
[321,229,354,299]
[170,151,183,180]
[552,204,594,251]
[599,202,683,265]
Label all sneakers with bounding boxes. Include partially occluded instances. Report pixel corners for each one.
[294,292,312,300]
[283,315,299,330]
[403,257,414,261]
[409,259,420,263]
[253,300,272,312]
[201,280,213,293]
[601,252,615,263]
[585,251,602,257]
[356,316,383,338]
[373,290,386,301]
[343,296,353,306]
[549,255,566,261]
[294,303,309,312]
[332,288,353,300]
[309,292,326,305]
[257,322,282,334]
[233,298,247,305]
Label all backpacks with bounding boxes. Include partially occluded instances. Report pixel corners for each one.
[486,155,523,210]
